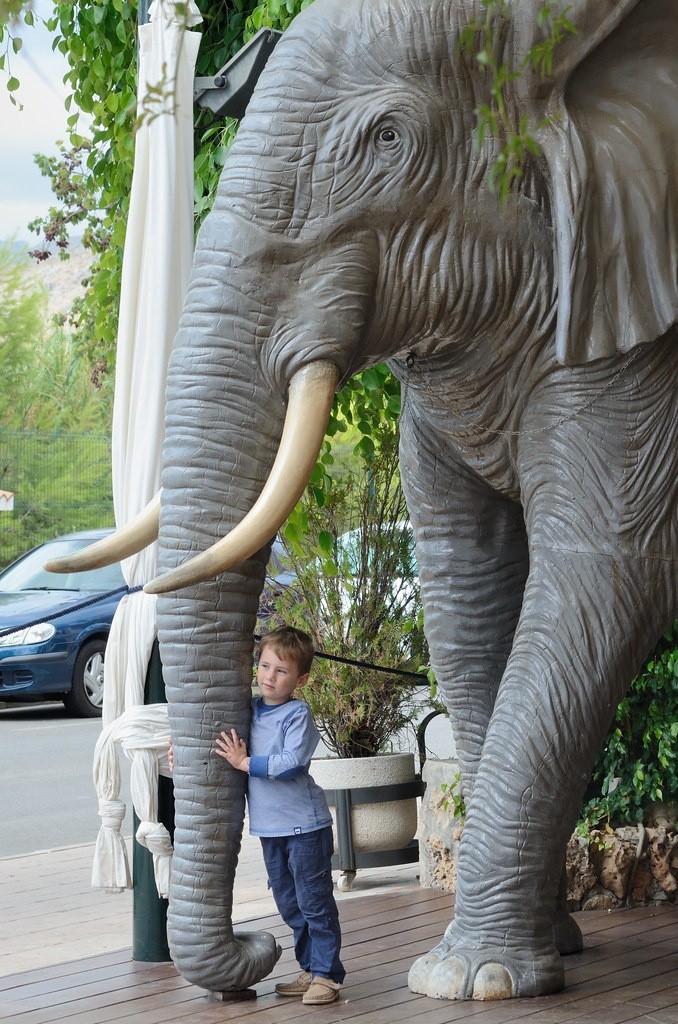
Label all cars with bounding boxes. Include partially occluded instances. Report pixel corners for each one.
[305,522,422,654]
[0,529,295,724]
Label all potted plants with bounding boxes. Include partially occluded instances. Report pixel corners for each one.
[248,362,448,855]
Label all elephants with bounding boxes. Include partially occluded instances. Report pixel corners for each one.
[44,2,676,1005]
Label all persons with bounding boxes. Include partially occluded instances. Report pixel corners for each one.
[168,628,345,1008]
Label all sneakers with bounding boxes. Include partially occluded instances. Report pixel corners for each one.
[275,972,312,994]
[303,977,341,1004]
